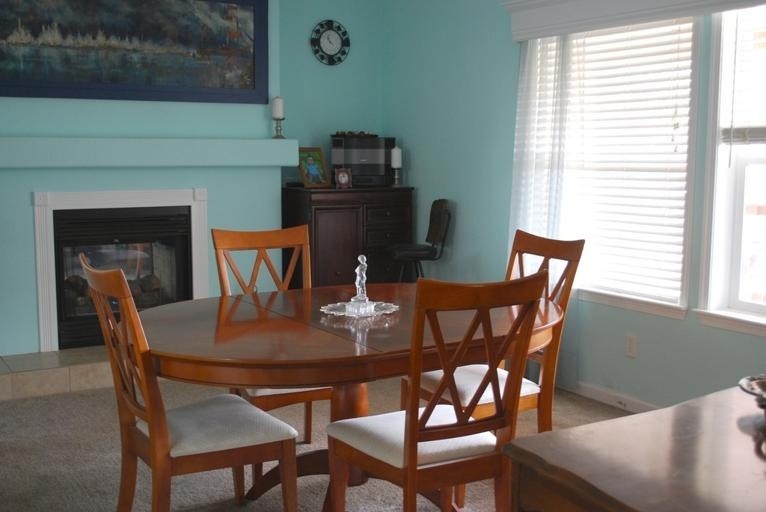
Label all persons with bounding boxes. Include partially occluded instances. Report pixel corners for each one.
[304,156,326,184]
[355,255,368,298]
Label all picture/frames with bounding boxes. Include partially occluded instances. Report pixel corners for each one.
[0,0,269,105]
[299,147,332,189]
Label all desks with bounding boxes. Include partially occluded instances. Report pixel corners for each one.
[501,376,765,512]
[112,282,566,512]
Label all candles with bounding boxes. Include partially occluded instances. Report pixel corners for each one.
[391,146,402,169]
[271,96,284,120]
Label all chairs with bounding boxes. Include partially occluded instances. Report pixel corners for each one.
[325,267,550,512]
[210,224,333,504]
[400,227,587,509]
[126,243,152,281]
[390,198,452,282]
[78,250,298,512]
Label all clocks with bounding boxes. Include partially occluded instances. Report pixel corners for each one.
[311,19,351,65]
[334,167,353,189]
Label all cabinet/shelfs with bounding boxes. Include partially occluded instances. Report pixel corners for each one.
[282,185,414,289]
